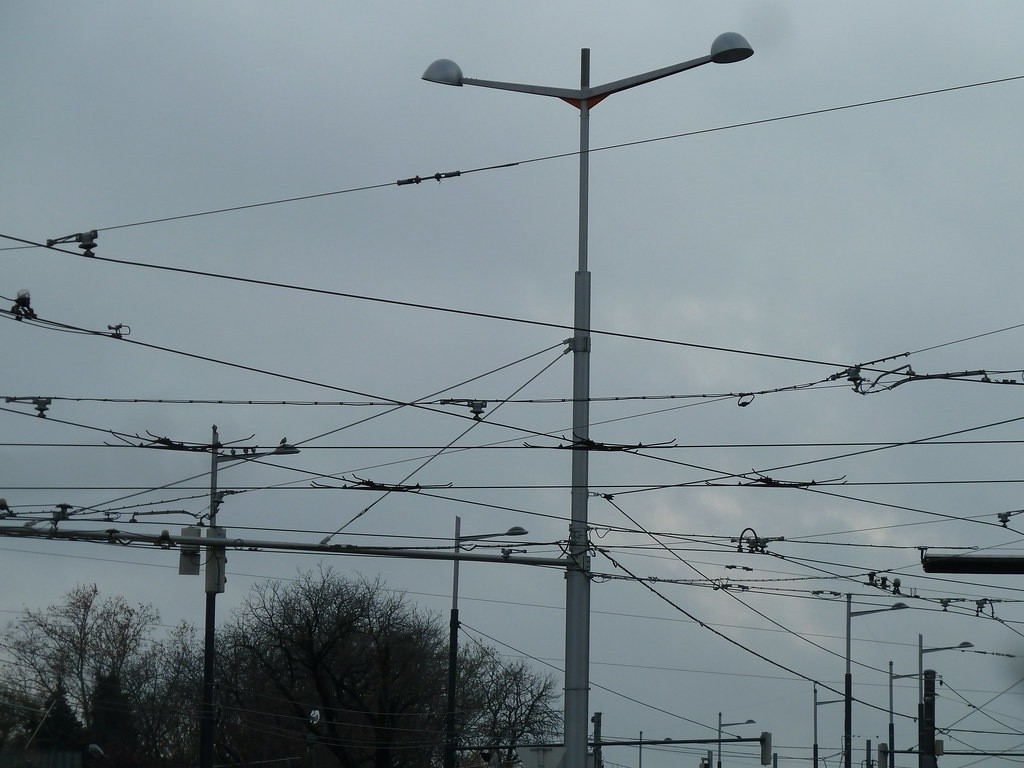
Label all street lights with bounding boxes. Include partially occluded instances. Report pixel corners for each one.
[442,515,528,767]
[810,687,857,768]
[198,423,305,768]
[917,632,976,768]
[716,710,756,768]
[418,31,756,768]
[839,593,913,767]
